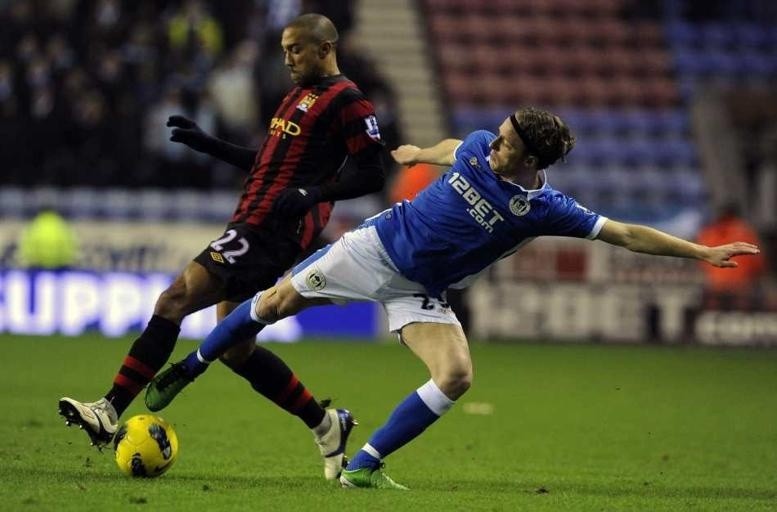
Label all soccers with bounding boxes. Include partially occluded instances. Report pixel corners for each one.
[115,415,178,478]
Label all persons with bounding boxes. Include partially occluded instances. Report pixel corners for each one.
[691,195,767,313]
[144,107,762,492]
[55,13,388,482]
[694,66,775,278]
[1,1,403,196]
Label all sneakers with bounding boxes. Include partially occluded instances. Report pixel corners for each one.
[57,396,120,451]
[145,362,193,412]
[314,407,360,479]
[340,461,410,491]
